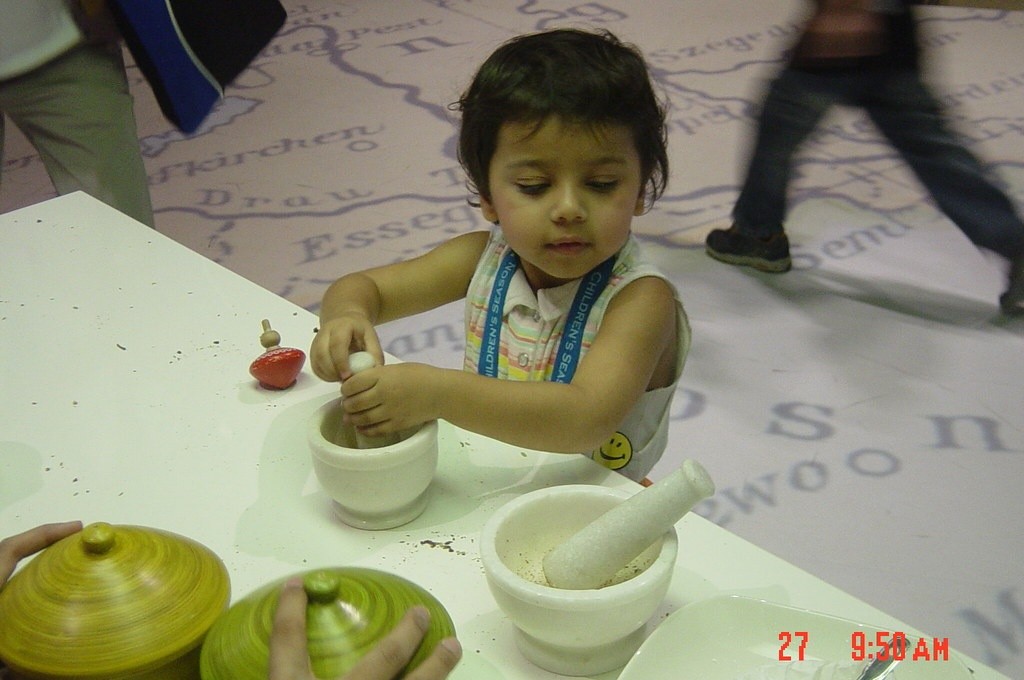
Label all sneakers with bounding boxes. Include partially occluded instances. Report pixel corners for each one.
[1000,259,1023,314]
[706,220,792,274]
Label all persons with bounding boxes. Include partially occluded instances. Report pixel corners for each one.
[1,520,463,680]
[705,0,1024,319]
[310,28,692,485]
[1,0,156,230]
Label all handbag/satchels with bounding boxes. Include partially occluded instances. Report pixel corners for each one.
[109,0,289,135]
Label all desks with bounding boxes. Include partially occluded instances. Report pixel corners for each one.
[0,190,1008,680]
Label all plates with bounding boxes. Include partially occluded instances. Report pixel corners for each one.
[616,593,974,680]
